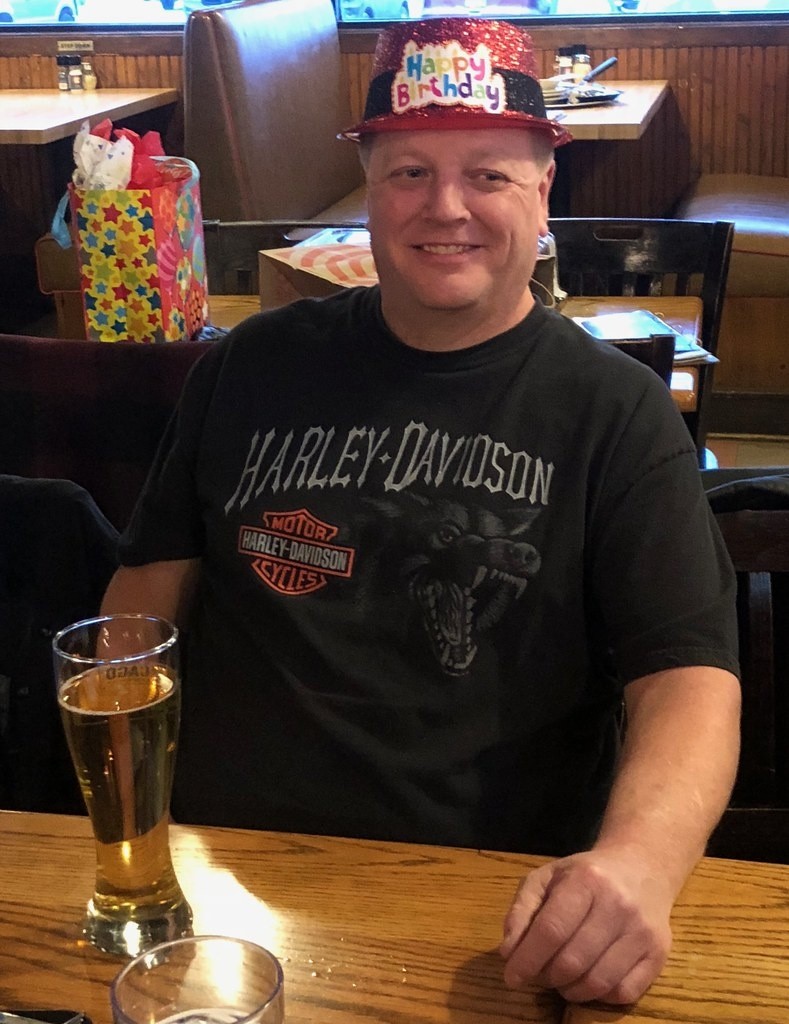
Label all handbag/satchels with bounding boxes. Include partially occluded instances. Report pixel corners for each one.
[68,156,209,344]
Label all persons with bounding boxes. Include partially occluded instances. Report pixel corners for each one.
[97,16,743,1009]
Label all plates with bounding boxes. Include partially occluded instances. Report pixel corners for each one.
[543,100,616,108]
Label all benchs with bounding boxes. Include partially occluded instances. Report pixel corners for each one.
[185,1,369,295]
[657,173,789,299]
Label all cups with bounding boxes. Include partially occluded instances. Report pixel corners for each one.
[112,935,284,1024]
[54,612,194,957]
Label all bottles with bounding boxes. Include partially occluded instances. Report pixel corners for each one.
[554,44,592,75]
[56,55,97,91]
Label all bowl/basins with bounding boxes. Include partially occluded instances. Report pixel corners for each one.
[540,79,560,90]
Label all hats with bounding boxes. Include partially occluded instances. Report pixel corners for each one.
[336,17,574,148]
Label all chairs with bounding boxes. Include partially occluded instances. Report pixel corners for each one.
[541,219,789,864]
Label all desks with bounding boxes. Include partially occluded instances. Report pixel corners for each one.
[538,80,670,219]
[0,810,789,1024]
[207,295,703,471]
[0,87,178,224]
